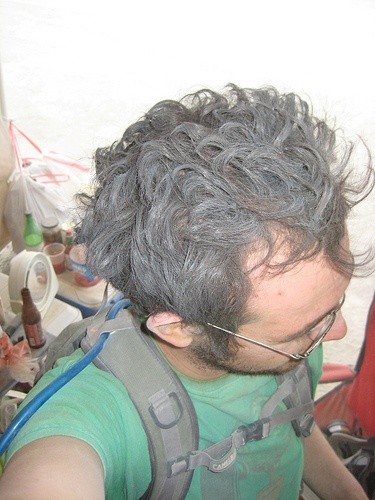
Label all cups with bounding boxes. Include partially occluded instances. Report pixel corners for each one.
[43,243,66,274]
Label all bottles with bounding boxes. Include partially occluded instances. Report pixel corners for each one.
[24,211,44,253]
[21,288,47,349]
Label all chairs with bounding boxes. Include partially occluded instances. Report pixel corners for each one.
[313,293,375,439]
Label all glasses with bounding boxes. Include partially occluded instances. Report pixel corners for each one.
[195,288,347,361]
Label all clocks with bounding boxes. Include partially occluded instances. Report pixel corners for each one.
[8,249,59,321]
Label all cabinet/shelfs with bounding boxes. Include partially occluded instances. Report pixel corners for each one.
[0,273,83,435]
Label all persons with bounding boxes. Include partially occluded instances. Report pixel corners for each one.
[1,83,374,500]
